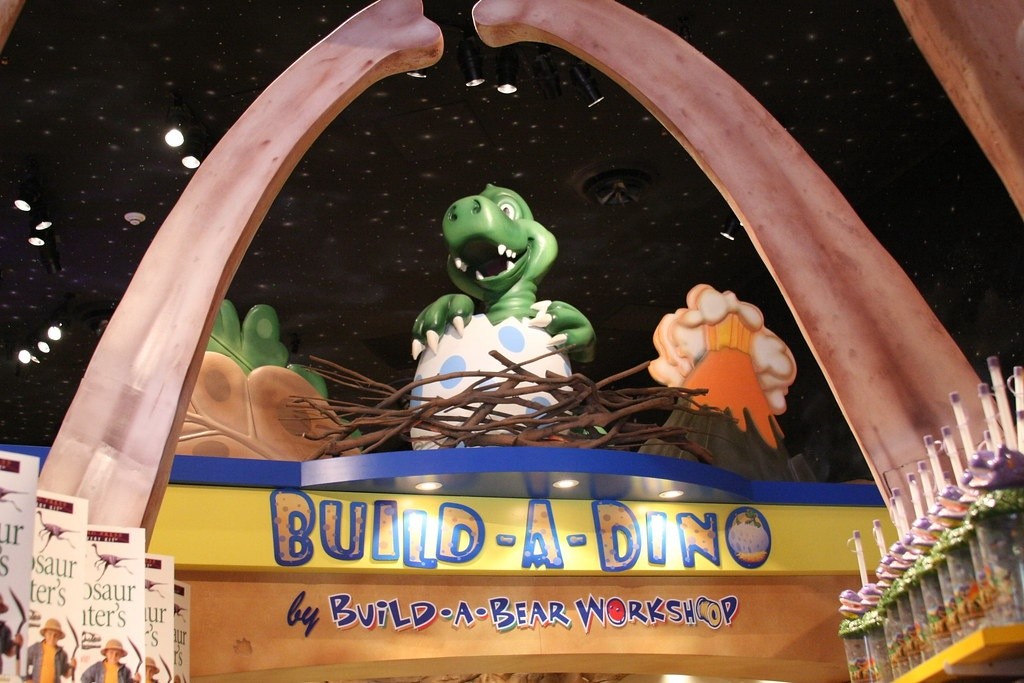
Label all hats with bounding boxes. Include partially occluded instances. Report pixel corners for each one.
[40,620,65,639]
[101,641,128,657]
[145,656,160,674]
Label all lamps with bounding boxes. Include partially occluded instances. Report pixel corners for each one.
[11,314,70,373]
[718,211,741,243]
[404,32,606,113]
[158,95,215,170]
[12,172,67,276]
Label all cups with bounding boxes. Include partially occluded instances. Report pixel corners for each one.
[841,513,1024,683]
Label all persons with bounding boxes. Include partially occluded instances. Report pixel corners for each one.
[0,593,24,672]
[145,657,160,683]
[21,619,77,683]
[81,640,142,683]
